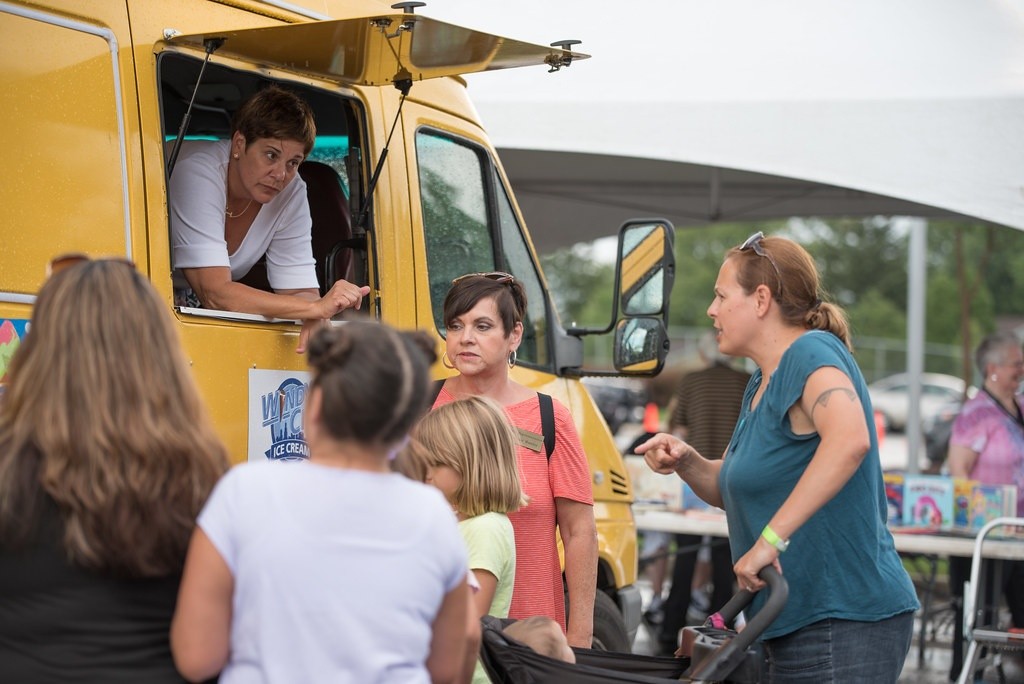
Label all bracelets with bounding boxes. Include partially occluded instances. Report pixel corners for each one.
[762,525,786,552]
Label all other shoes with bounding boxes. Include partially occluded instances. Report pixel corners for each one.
[646,608,666,625]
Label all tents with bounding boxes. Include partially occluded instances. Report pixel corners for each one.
[476,98,1024,471]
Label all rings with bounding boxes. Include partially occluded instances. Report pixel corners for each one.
[746,586,752,591]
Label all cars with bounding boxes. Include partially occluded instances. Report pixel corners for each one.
[868,373,979,437]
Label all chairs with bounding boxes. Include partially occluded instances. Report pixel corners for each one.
[954,518,1024,684]
[297,160,358,306]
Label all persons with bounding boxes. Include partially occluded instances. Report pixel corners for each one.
[0,257,232,684]
[503,615,576,664]
[409,394,521,684]
[170,320,482,684]
[654,333,751,657]
[425,271,598,650]
[948,332,1024,684]
[635,232,921,684]
[168,87,371,353]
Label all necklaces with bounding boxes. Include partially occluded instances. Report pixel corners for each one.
[225,199,253,218]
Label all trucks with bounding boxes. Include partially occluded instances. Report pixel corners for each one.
[0,0,678,654]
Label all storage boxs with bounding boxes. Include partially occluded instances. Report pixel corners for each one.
[881,472,1017,527]
[626,454,706,510]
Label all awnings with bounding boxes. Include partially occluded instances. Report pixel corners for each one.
[167,14,591,214]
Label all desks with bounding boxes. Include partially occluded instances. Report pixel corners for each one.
[635,507,1024,668]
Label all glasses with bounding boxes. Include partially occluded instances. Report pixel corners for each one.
[47,254,136,279]
[453,272,516,301]
[739,231,781,287]
